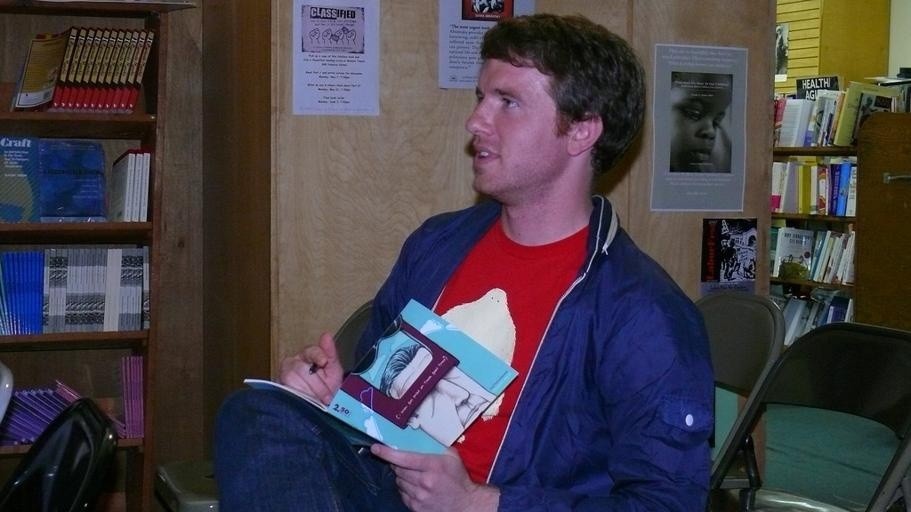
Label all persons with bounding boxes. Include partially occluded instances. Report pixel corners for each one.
[671,72,733,175]
[209,12,715,511]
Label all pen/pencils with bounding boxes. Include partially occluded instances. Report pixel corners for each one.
[310,364,320,376]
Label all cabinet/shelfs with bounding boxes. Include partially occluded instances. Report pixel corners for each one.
[768,110,910,350]
[0,1,194,511]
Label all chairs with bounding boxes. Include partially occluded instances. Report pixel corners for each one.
[701,319,909,512]
[0,398,121,511]
[690,286,786,512]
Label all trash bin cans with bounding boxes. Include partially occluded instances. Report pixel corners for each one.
[155,460,219,512]
